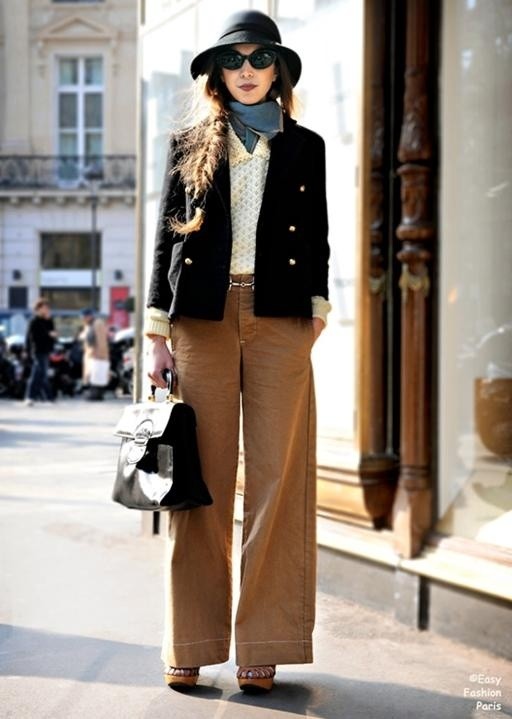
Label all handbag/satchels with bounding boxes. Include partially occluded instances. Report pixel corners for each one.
[111,399,211,512]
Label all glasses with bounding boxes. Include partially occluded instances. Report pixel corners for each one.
[216,49,276,70]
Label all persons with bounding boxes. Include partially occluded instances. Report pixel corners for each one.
[142,8,334,697]
[78,308,114,402]
[20,299,61,406]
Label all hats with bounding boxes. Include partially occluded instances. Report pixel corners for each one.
[190,10,302,86]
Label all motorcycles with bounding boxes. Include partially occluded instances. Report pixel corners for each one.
[0,326,136,403]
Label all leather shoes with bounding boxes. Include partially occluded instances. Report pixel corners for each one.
[236,664,276,692]
[164,664,199,690]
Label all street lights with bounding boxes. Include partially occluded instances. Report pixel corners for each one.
[81,160,104,319]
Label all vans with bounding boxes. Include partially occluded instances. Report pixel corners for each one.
[1,308,112,337]
